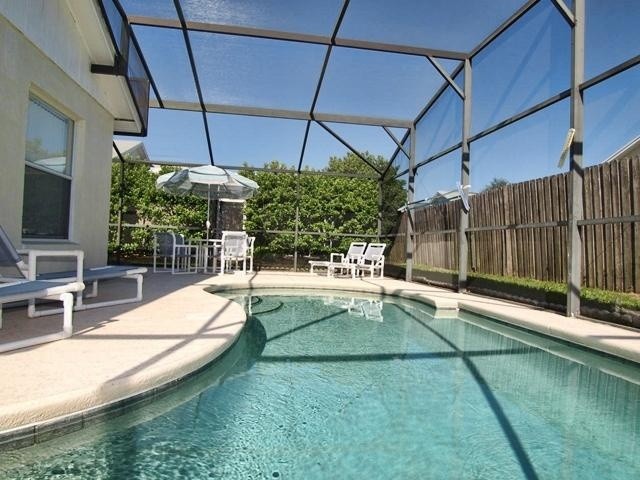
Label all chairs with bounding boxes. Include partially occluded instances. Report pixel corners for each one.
[152,227,387,282]
[0,223,149,319]
[1,275,87,355]
[322,297,385,323]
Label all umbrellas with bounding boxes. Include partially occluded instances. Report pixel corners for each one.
[156,164,260,266]
